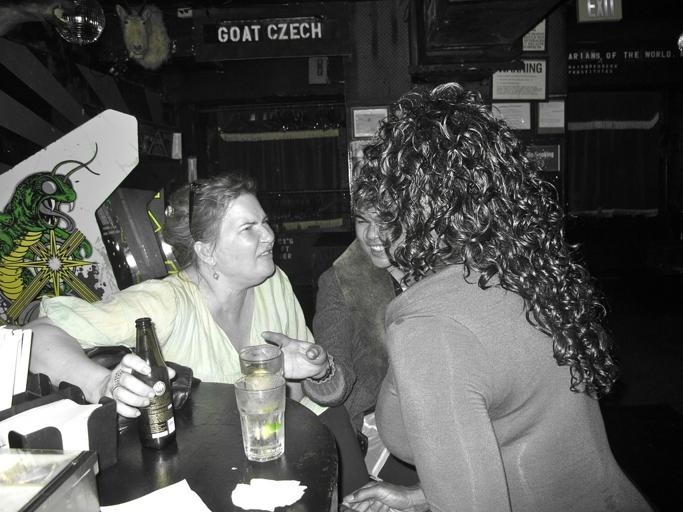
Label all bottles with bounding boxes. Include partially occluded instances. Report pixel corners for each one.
[134,316,177,451]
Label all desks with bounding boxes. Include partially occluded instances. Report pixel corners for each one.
[92,380,341,512]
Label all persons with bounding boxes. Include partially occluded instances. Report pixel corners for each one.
[339,81,653,511]
[17,169,368,501]
[249,175,419,488]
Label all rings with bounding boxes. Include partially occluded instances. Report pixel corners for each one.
[114,369,123,381]
[109,384,121,396]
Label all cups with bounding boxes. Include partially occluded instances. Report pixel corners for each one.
[232,372,287,463]
[238,343,284,379]
